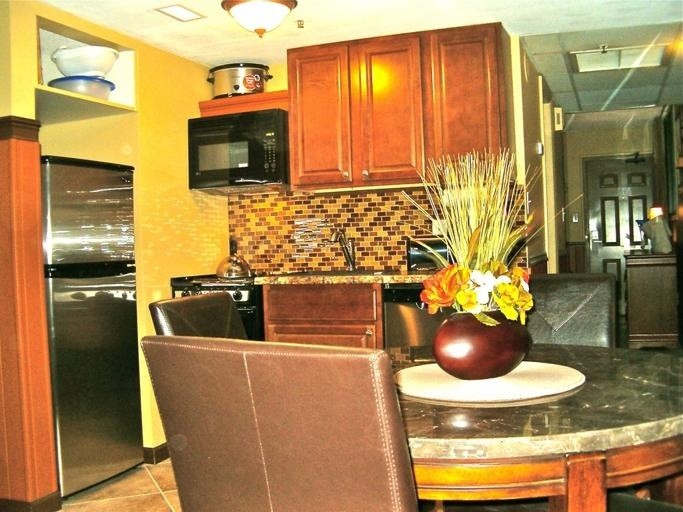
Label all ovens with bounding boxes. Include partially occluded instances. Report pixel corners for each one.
[170,285,263,341]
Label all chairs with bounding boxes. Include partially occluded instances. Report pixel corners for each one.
[147,291,250,341]
[526,272,618,347]
[137,335,419,512]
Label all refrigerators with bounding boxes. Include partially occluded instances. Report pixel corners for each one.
[39,154,145,501]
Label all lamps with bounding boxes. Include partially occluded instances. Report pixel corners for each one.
[219,0,301,41]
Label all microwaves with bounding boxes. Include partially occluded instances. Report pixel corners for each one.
[186,108,289,194]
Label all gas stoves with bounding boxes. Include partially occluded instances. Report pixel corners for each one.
[180,277,255,284]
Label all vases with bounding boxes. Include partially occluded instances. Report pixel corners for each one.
[432,309,532,381]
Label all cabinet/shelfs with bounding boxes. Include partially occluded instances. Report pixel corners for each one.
[624,252,681,350]
[288,31,424,190]
[424,22,515,179]
[262,283,384,350]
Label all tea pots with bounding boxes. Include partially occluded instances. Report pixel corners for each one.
[216,239,254,281]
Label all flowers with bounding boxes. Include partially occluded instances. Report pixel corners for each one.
[397,145,587,324]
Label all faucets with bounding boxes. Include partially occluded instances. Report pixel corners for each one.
[328,230,355,272]
